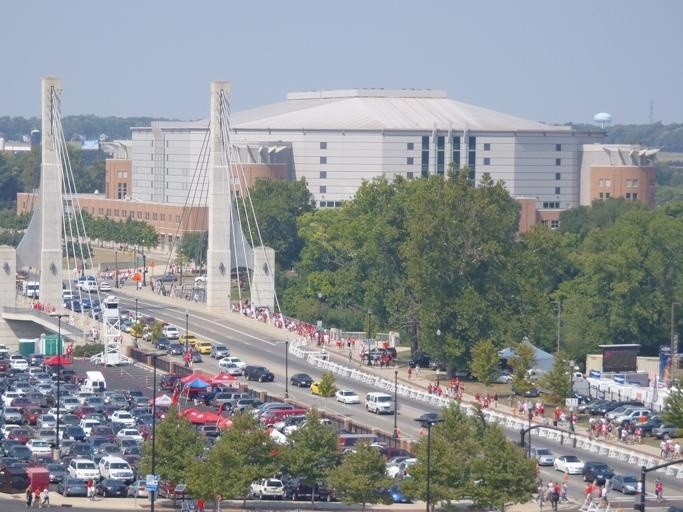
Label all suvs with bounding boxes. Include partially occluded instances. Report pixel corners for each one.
[582,462,616,486]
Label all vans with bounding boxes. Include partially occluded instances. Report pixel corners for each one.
[410,351,429,368]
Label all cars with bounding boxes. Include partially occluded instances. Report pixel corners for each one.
[428,360,446,372]
[530,446,555,467]
[610,474,640,495]
[554,455,585,476]
[417,413,444,428]
[461,367,682,441]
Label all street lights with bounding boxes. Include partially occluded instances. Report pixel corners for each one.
[668,301,680,386]
[434,327,440,389]
[550,301,561,365]
[518,425,600,450]
[114,247,118,288]
[119,246,147,287]
[141,352,166,512]
[414,419,445,512]
[569,360,573,399]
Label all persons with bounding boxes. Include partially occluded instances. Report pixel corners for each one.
[197,498,205,512]
[24,484,51,509]
[426,375,683,511]
[86,479,98,501]
[28,300,57,314]
[100,268,144,290]
[231,298,421,379]
